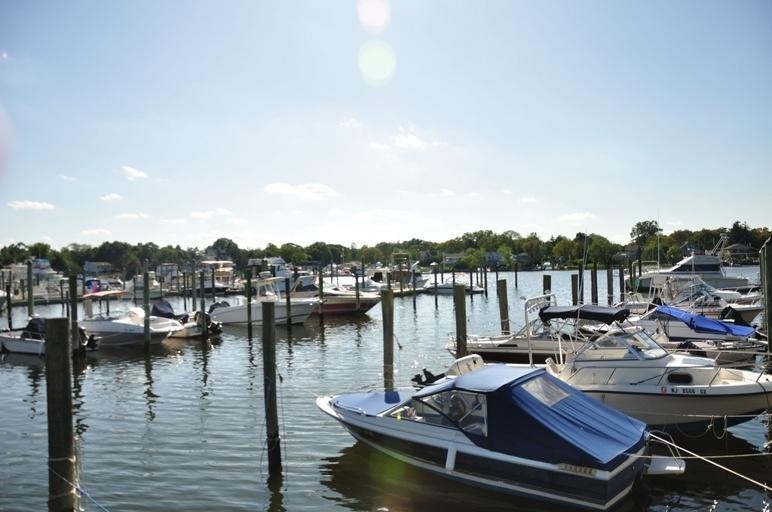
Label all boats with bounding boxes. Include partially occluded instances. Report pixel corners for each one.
[616,239,767,374]
[151,300,225,339]
[206,278,327,328]
[410,292,772,448]
[316,354,687,512]
[339,258,487,297]
[0,316,99,356]
[79,289,184,347]
[282,275,384,317]
